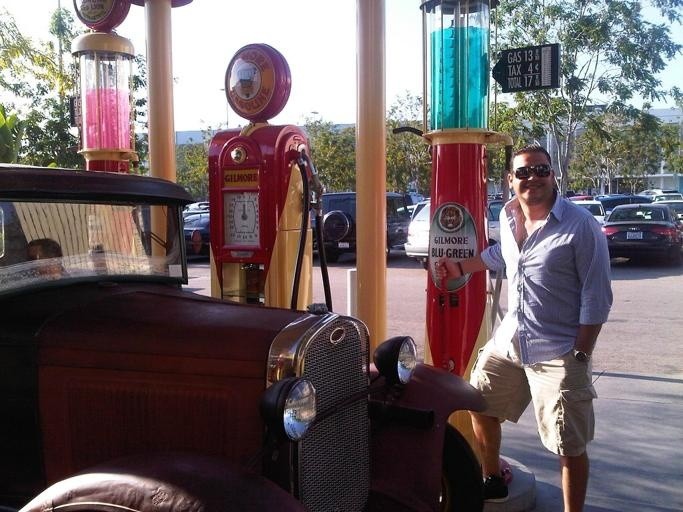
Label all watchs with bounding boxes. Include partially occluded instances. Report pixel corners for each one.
[571,348,591,363]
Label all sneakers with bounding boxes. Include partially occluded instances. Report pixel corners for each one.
[484,474,509,503]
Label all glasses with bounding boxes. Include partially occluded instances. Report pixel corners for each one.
[512,163,551,181]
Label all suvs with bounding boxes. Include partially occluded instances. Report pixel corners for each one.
[321,188,410,265]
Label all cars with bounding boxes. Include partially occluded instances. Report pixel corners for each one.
[181,201,210,258]
[405,187,683,271]
[1,163,488,507]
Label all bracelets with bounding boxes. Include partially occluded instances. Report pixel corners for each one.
[456,261,466,277]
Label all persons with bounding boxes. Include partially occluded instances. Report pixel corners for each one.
[21,237,72,282]
[432,146,614,512]
